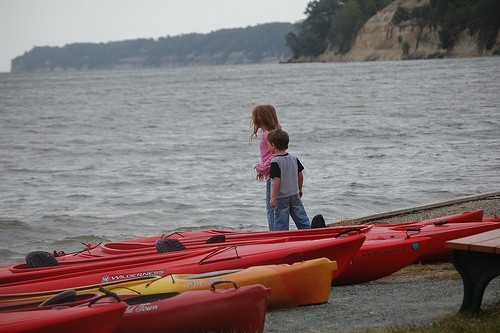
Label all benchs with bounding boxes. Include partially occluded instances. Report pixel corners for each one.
[445,228,500,316]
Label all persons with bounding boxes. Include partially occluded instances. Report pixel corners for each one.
[267,128,312,231]
[248,105,283,231]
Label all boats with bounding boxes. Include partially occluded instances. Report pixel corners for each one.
[0,208,500,308]
[0,301,128,333]
[0,278,272,333]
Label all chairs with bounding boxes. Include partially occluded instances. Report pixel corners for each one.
[156,238,184,253]
[25,250,58,268]
[39,289,77,307]
[205,234,225,243]
[311,215,326,228]
